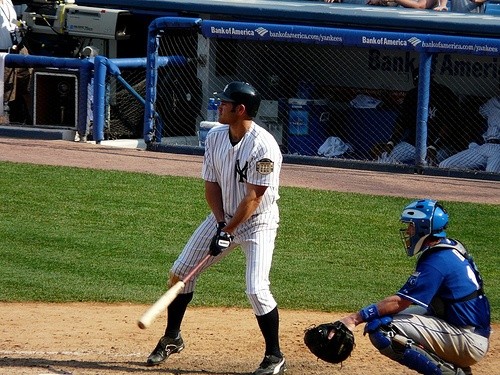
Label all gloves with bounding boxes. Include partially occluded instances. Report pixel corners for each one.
[209,230,234,256]
[214,222,227,232]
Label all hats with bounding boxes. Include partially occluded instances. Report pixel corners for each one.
[213,81,262,114]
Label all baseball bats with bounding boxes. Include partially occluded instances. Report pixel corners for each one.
[138,254,212,329]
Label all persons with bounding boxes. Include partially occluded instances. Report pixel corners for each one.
[373,57,456,167]
[433,0,487,13]
[304,199,490,375]
[0,0,20,126]
[365,0,440,9]
[147,81,286,375]
[439,90,500,174]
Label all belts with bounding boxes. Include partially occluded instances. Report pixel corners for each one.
[483,139,500,144]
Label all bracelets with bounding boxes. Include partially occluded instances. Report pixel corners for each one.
[359,304,379,322]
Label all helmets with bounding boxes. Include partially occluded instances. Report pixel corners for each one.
[398,199,449,257]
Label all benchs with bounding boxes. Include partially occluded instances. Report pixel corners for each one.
[338,109,415,164]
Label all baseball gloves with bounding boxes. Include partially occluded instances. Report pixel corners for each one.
[304,321,355,365]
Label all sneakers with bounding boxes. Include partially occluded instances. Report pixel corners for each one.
[147,331,185,365]
[251,352,286,375]
